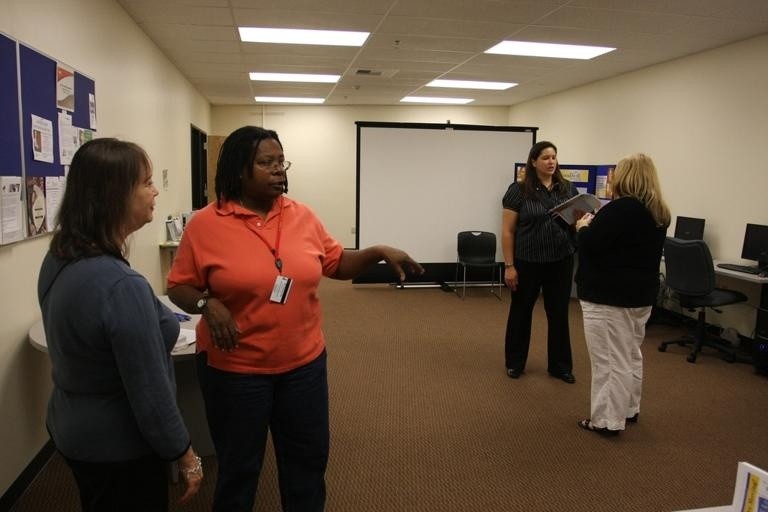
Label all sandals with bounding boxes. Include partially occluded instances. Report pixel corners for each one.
[579,418,620,439]
[625,413,638,423]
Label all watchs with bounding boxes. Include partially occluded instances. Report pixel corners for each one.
[196,296,211,313]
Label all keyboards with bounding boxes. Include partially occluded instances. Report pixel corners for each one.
[717,263,762,275]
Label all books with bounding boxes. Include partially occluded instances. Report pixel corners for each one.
[550,194,602,225]
[732,462,768,512]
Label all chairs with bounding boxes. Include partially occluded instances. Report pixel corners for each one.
[657,236,748,363]
[454,231,505,301]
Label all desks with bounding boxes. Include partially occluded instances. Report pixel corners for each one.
[158,240,180,296]
[28,295,203,483]
[653,256,768,340]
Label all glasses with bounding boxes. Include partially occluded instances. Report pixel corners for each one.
[255,160,291,171]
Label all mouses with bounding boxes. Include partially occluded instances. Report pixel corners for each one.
[759,270,768,277]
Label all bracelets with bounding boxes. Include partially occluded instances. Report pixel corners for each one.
[505,264,514,267]
[179,456,204,479]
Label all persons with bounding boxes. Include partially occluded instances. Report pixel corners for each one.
[166,126,426,512]
[37,138,205,512]
[502,141,580,383]
[575,153,671,437]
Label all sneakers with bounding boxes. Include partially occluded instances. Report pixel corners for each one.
[548,369,576,384]
[508,369,521,379]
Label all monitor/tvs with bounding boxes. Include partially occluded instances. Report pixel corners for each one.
[674,215,706,241]
[741,222,768,268]
